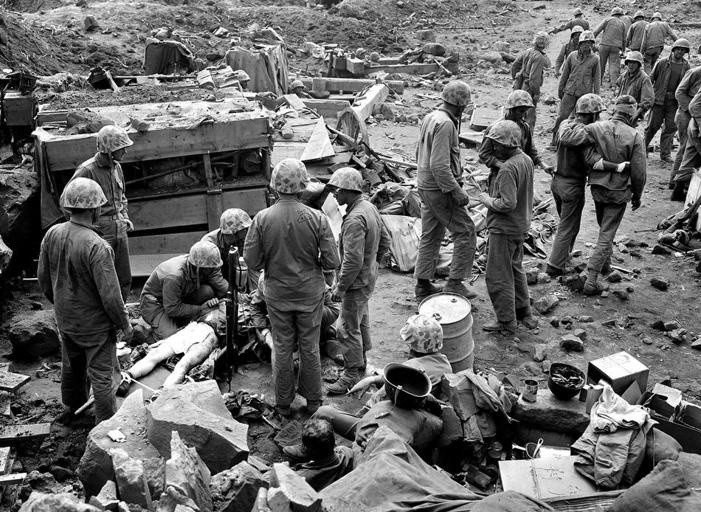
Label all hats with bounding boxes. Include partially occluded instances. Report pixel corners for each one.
[282,419,345,471]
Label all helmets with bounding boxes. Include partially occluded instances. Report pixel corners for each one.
[187,239,227,270]
[670,38,692,55]
[503,89,536,111]
[548,357,589,401]
[59,175,109,210]
[574,6,582,17]
[217,204,254,235]
[568,25,598,46]
[532,28,550,49]
[574,92,608,116]
[398,311,446,355]
[268,156,312,195]
[484,118,525,151]
[325,166,365,194]
[381,359,433,407]
[620,49,647,69]
[438,79,477,107]
[609,6,666,21]
[95,122,135,155]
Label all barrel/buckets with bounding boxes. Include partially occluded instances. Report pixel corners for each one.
[417,292,475,375]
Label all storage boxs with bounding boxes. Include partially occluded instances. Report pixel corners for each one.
[587,350,649,392]
[4,92,36,127]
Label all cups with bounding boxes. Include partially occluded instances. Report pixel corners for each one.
[522,379,539,403]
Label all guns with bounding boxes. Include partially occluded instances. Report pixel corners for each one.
[225,245,240,393]
[362,140,402,183]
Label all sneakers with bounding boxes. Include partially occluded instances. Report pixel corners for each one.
[516,306,531,319]
[323,369,364,396]
[270,401,295,418]
[441,276,479,300]
[544,264,564,278]
[306,394,325,413]
[413,277,442,300]
[480,314,520,334]
[334,353,349,367]
[584,269,611,295]
